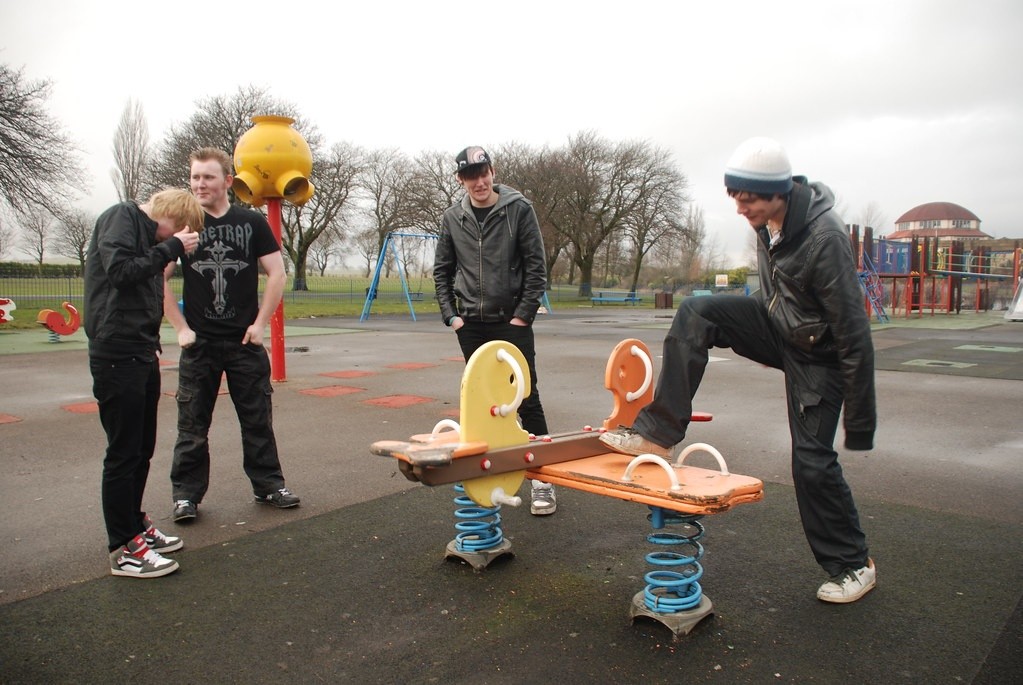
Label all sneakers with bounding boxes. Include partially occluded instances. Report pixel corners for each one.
[172,499,197,521]
[244,489,301,507]
[529,484,558,515]
[109,534,179,579]
[600,429,675,466]
[818,556,878,603]
[140,512,184,554]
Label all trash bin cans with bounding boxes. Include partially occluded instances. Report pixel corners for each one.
[655,291,675,309]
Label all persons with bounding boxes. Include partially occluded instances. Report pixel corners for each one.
[433,145,564,516]
[596,141,881,603]
[160,147,300,519]
[82,189,206,579]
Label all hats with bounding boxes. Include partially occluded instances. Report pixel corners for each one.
[452,146,489,174]
[725,142,795,194]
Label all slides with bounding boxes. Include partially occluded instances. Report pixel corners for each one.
[1003,278,1023,321]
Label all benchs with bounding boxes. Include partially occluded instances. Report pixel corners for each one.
[590,290,642,306]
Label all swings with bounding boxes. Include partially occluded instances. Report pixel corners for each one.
[400,236,427,301]
